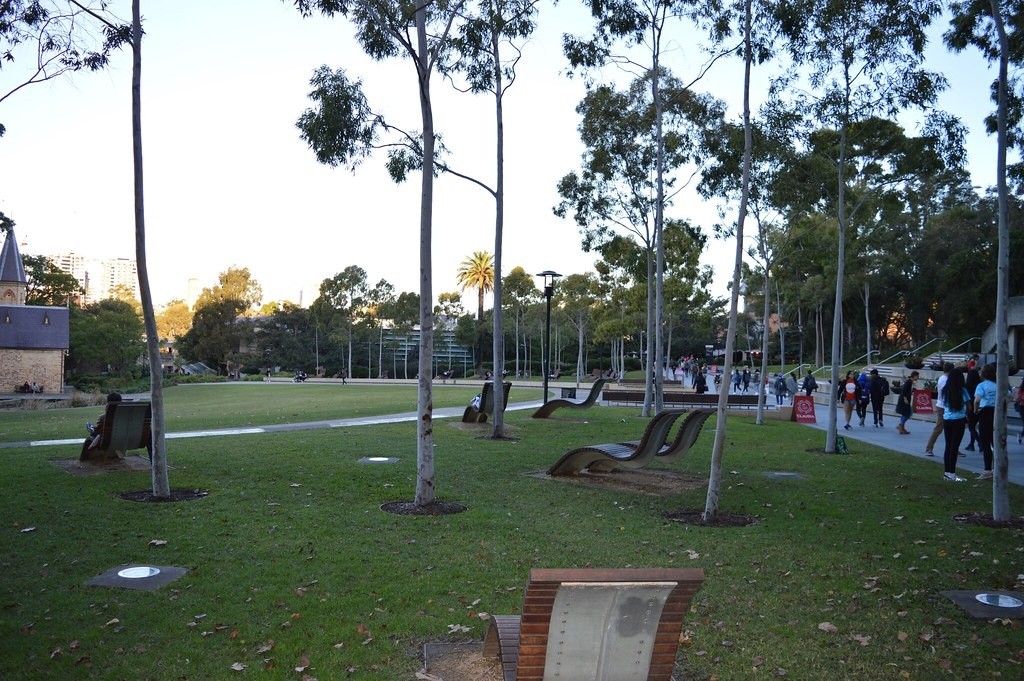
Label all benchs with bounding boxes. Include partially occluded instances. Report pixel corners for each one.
[14,389,44,394]
[603,391,766,409]
[618,379,685,388]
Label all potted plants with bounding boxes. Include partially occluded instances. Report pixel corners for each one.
[889,380,903,394]
[923,380,938,399]
[903,354,925,369]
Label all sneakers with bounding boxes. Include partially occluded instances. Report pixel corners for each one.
[858,417,865,426]
[965,444,976,451]
[924,449,934,456]
[899,430,910,434]
[896,423,906,433]
[957,451,966,457]
[879,420,884,427]
[974,470,993,480]
[1017,431,1022,445]
[872,424,878,428]
[943,475,968,482]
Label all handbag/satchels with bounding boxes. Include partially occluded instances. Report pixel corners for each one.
[703,386,709,391]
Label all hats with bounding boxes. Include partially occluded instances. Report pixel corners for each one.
[778,372,784,379]
[735,369,739,371]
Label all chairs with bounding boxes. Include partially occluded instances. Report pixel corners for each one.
[434,369,455,380]
[590,368,628,383]
[533,377,605,418]
[376,370,389,379]
[79,400,152,462]
[547,370,560,382]
[462,382,513,423]
[483,568,705,681]
[546,408,715,478]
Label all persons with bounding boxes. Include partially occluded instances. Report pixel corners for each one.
[837,370,859,407]
[856,372,872,427]
[86,393,122,437]
[786,371,798,405]
[936,368,972,481]
[773,373,787,405]
[968,354,1024,479]
[837,370,861,430]
[895,371,919,434]
[23,381,39,394]
[265,368,522,385]
[870,369,884,428]
[801,370,819,396]
[653,352,768,394]
[924,363,966,457]
[964,370,982,453]
[602,368,617,381]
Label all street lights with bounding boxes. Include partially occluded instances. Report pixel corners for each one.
[735,330,739,368]
[535,270,563,405]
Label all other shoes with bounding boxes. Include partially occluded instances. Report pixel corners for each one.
[844,424,852,430]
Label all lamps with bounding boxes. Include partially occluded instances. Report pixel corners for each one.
[42,311,51,326]
[63,349,70,356]
[2,310,13,325]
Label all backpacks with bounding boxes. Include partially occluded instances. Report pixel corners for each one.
[879,380,890,397]
[805,376,815,391]
[714,375,720,384]
